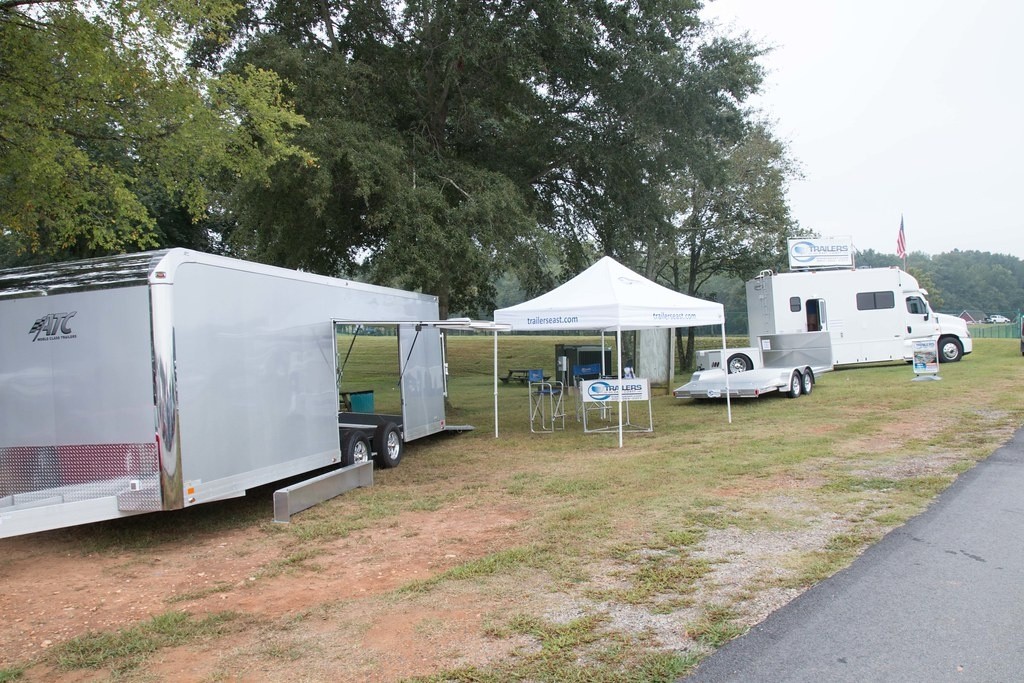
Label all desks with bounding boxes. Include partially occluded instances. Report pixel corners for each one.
[339,389,374,413]
[507,369,529,385]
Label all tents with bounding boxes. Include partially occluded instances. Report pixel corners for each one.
[493,256,732,448]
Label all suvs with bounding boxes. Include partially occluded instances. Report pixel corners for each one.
[987,313,1011,325]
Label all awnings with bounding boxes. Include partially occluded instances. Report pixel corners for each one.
[332,317,512,389]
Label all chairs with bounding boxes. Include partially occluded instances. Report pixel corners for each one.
[529,369,566,433]
[573,364,612,423]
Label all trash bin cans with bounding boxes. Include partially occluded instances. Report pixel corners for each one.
[348,389,375,413]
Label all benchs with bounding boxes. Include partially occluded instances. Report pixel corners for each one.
[499,376,551,385]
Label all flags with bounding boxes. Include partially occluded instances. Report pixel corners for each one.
[896,214,906,260]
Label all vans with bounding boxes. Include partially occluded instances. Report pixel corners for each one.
[744,264,973,377]
